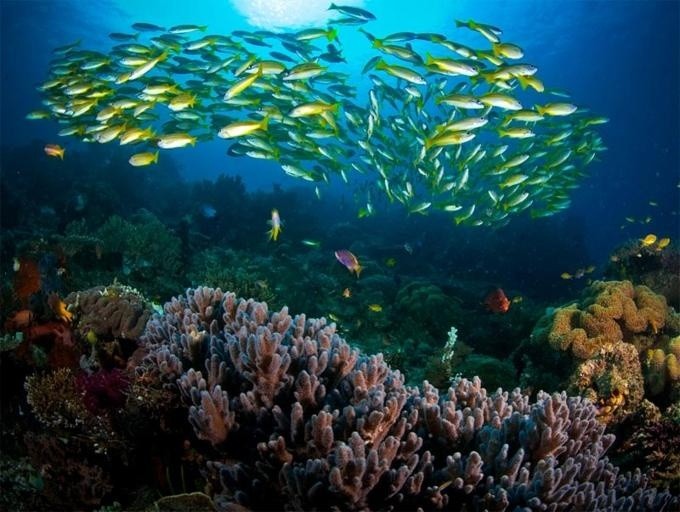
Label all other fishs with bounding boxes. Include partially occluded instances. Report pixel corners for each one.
[13,18,671,344]
